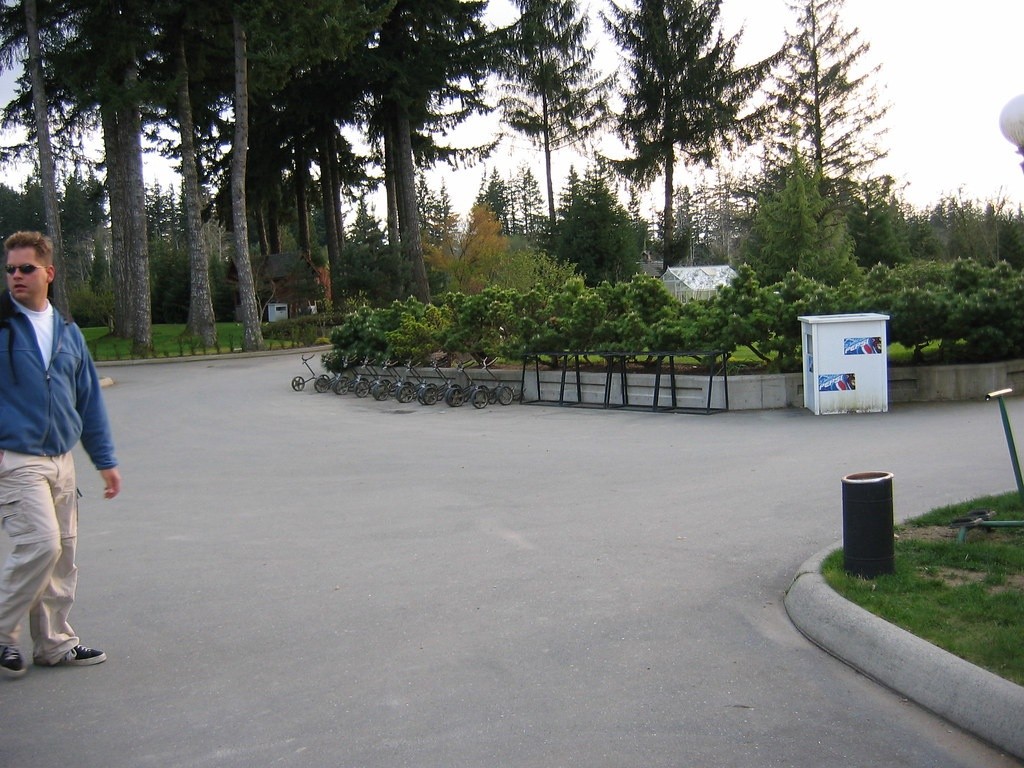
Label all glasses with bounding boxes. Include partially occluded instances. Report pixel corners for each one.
[5,264,47,274]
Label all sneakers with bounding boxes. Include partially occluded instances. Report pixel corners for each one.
[34,644,106,666]
[0,645,26,676]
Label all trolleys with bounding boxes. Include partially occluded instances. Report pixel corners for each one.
[289,353,514,409]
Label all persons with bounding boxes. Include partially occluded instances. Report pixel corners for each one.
[0,232,119,678]
